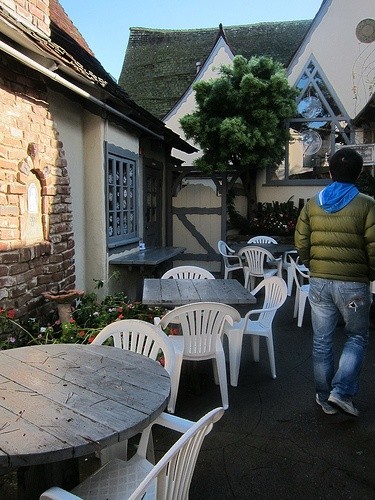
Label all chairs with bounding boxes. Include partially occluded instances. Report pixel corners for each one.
[26,234,310,500]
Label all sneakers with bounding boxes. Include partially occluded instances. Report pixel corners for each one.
[316,393,337,414]
[328,394,360,417]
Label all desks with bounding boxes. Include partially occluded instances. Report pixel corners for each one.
[141,277,257,307]
[109,245,184,273]
[229,243,295,255]
[0,341,171,500]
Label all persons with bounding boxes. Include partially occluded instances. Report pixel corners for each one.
[294,148,375,418]
